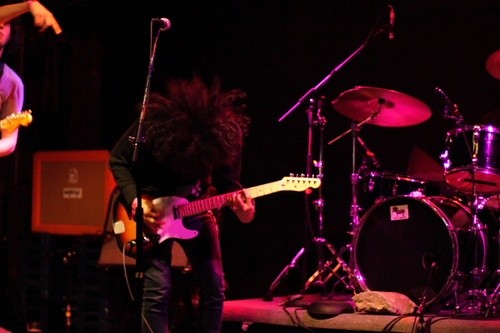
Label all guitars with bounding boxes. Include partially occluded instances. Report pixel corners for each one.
[113,172,325,257]
[0,108,33,132]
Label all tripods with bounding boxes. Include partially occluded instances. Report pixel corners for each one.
[266,25,386,302]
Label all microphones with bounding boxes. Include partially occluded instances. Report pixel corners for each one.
[388,8,396,39]
[152,17,172,30]
[357,137,382,168]
[128,235,160,247]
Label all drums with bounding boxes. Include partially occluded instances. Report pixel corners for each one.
[354,196,488,309]
[443,123,500,192]
[356,171,441,217]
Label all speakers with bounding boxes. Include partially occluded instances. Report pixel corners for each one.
[31,150,119,235]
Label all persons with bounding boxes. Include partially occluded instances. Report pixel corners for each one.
[0,0,63,157]
[109,77,255,333]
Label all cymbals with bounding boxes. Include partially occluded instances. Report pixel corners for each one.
[331,85,433,128]
